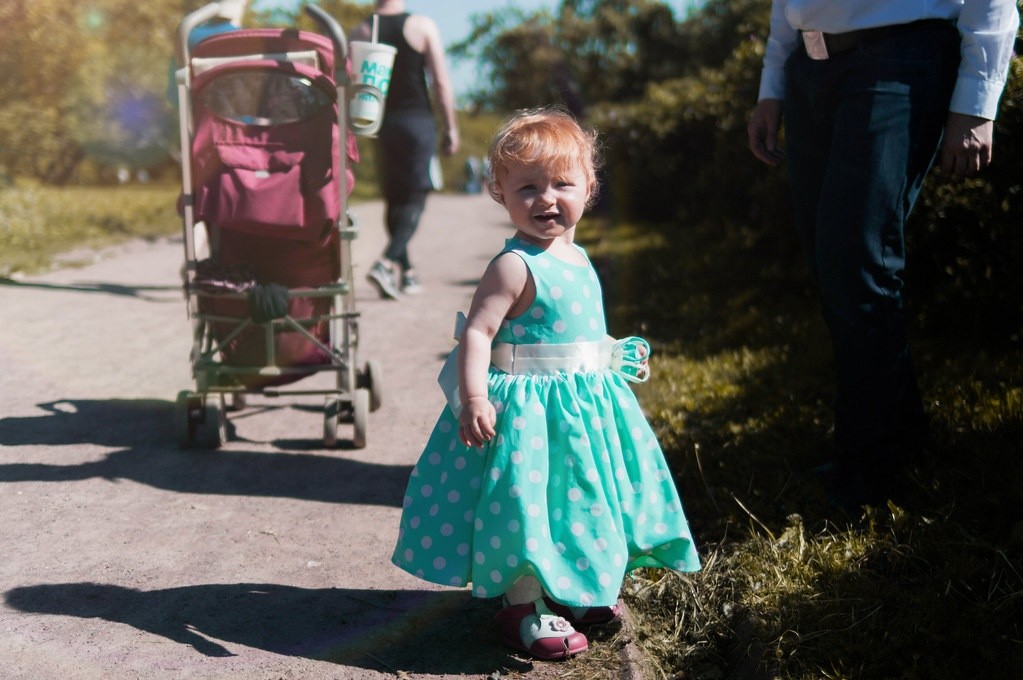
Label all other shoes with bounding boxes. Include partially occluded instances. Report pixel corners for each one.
[401,273,419,294]
[366,259,400,301]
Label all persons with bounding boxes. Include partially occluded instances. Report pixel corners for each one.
[361,0,459,299]
[747,0,1020,534]
[390,113,701,659]
[465,156,490,192]
[167,0,241,113]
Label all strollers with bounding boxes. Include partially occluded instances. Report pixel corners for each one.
[174,0,382,450]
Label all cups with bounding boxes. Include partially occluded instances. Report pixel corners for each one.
[347,41,398,136]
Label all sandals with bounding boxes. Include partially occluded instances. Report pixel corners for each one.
[547,594,622,623]
[495,588,589,658]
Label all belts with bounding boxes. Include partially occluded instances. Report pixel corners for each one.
[796,18,957,61]
